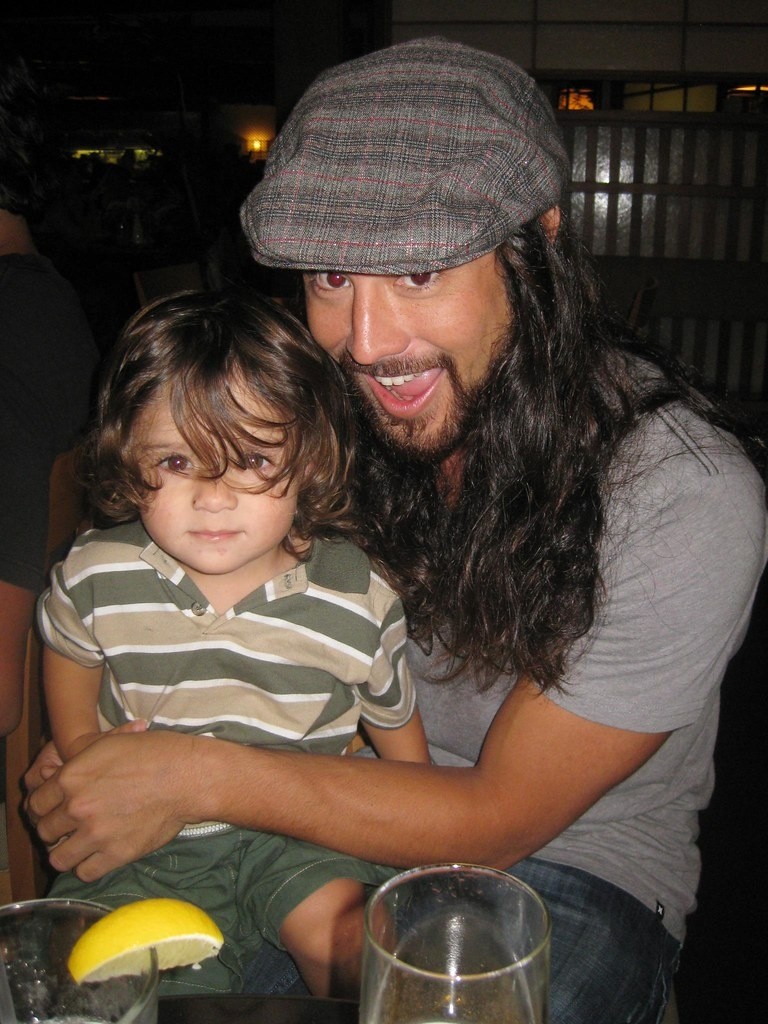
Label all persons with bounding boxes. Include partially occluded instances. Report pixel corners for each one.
[34,284,436,1000]
[19,38,767,1024]
[0,153,101,589]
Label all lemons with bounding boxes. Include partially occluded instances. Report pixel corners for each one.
[67,897,225,986]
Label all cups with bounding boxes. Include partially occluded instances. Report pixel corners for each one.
[361,861,551,1024]
[0,899,159,1024]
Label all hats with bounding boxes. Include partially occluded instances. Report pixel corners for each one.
[240,39,572,273]
